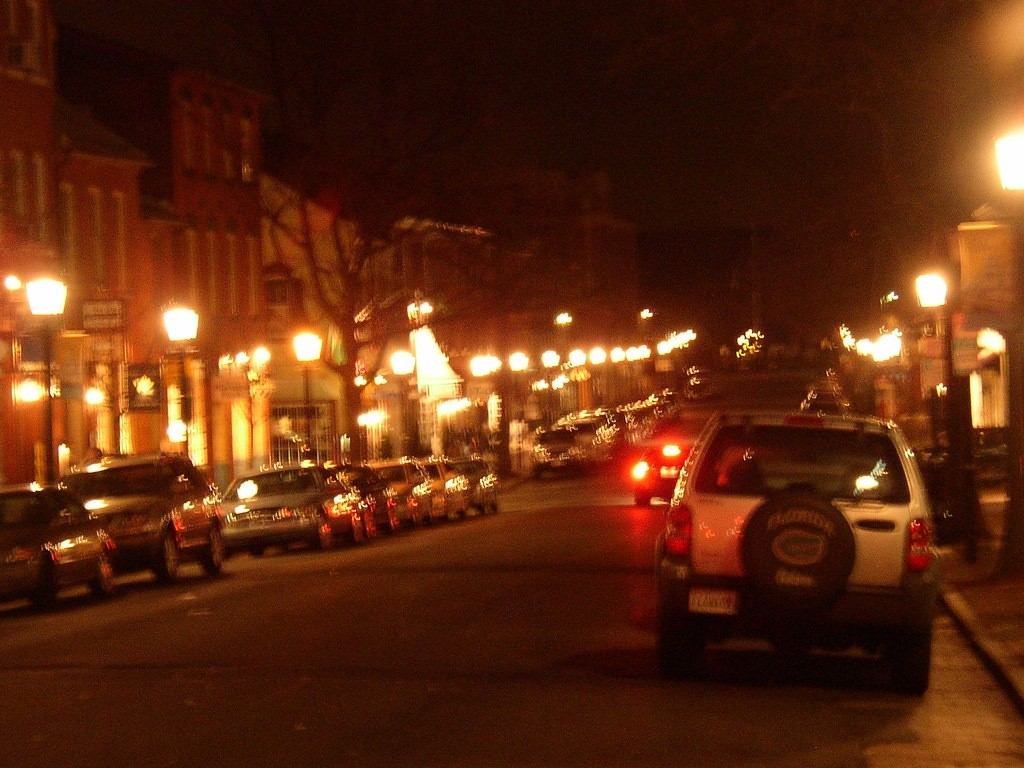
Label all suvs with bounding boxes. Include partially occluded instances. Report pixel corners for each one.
[652,407,952,698]
[58,450,225,585]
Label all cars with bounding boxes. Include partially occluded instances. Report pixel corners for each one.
[218,455,500,562]
[0,484,119,610]
[523,362,717,472]
[634,434,698,506]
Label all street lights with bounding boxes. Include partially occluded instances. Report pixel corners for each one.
[21,271,67,480]
[293,329,325,460]
[163,306,200,457]
